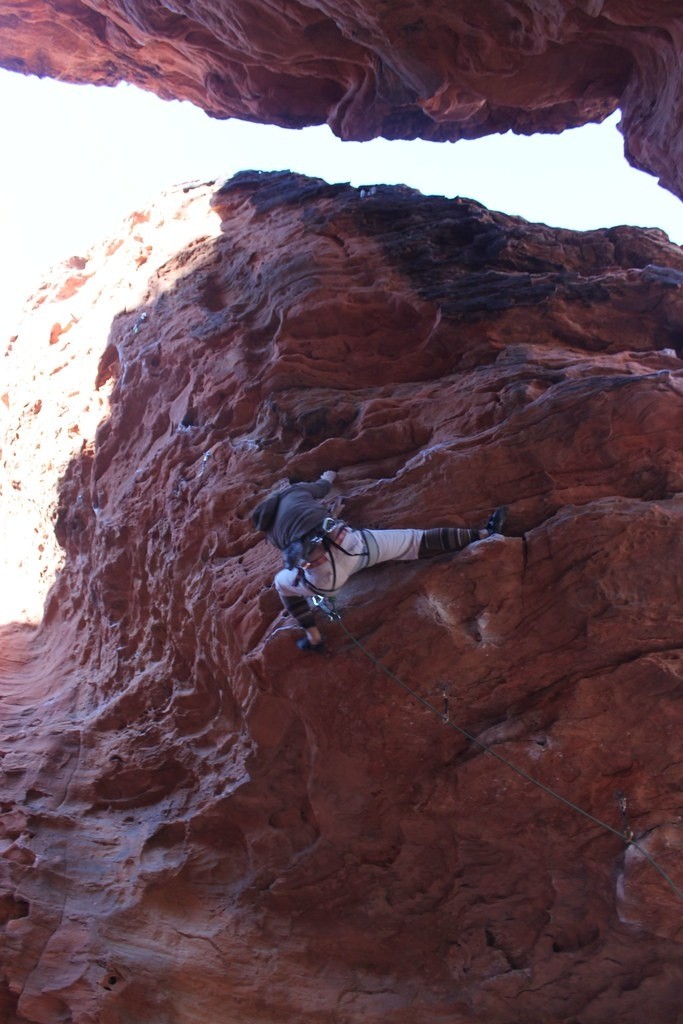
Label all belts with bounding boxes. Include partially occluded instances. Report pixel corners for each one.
[304,530,346,569]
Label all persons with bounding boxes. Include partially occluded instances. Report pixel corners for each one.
[250,471,507,653]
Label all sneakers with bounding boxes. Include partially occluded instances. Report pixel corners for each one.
[486,506,506,535]
[297,637,325,653]
